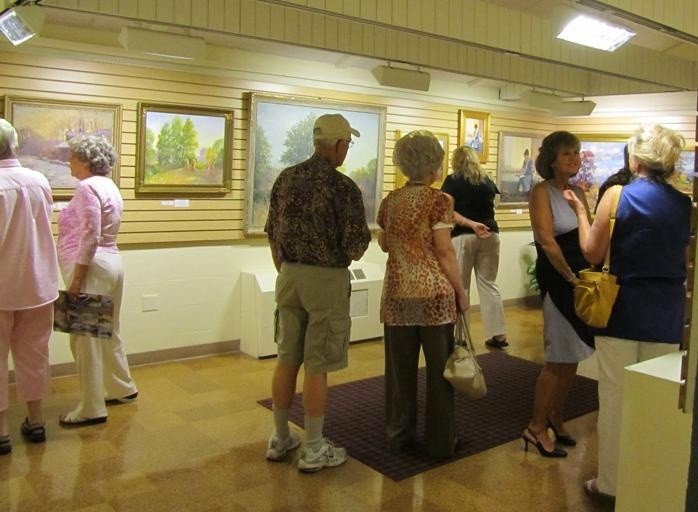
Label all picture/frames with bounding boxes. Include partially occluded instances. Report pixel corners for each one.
[665,141,698,196]
[245,92,389,237]
[458,108,490,164]
[494,130,545,210]
[3,93,122,201]
[569,132,631,229]
[394,129,450,190]
[134,100,235,195]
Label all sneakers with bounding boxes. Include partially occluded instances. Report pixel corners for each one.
[266,428,302,462]
[298,444,348,473]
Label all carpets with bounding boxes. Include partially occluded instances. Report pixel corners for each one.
[256,350,601,482]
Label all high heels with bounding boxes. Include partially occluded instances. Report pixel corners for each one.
[521,427,568,458]
[548,417,576,446]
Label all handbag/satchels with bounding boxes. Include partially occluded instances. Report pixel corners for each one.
[574,268,622,329]
[443,345,490,400]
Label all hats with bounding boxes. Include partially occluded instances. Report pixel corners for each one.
[314,114,360,140]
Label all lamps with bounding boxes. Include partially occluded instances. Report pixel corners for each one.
[549,3,639,52]
[372,61,431,92]
[117,22,210,63]
[549,94,596,116]
[0,3,48,47]
[520,86,563,110]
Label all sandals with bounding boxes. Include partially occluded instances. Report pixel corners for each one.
[59,413,108,428]
[485,336,511,350]
[21,416,45,443]
[104,391,138,404]
[0,433,11,455]
[585,479,615,511]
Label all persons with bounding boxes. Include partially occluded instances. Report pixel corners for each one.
[56,134,138,427]
[469,122,483,151]
[516,148,531,198]
[593,144,637,215]
[0,118,60,455]
[440,146,510,350]
[376,130,469,464]
[520,131,603,458]
[564,126,689,508]
[264,114,372,474]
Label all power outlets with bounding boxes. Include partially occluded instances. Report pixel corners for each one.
[143,294,157,311]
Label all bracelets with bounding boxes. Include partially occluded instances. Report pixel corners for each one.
[566,273,576,283]
[460,217,465,227]
